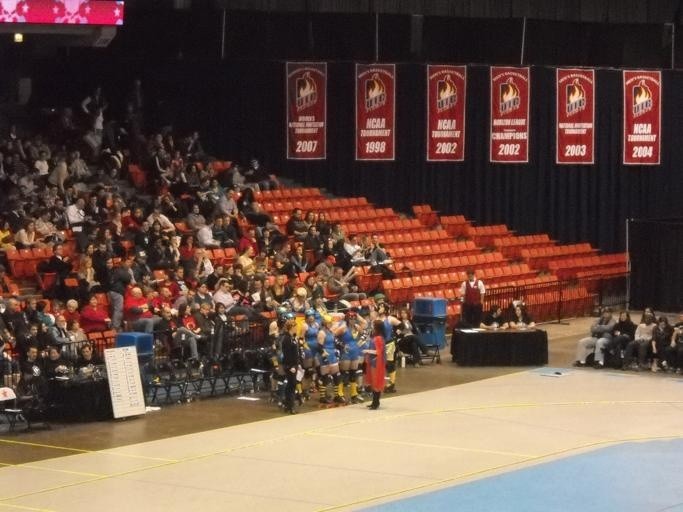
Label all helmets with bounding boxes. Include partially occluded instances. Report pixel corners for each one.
[374,293,389,312]
[277,301,357,325]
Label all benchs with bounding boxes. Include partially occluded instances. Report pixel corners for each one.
[1,158,633,415]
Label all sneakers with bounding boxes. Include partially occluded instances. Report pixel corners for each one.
[271,384,396,410]
[575,360,683,375]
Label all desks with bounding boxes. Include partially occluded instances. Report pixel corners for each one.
[452,323,553,364]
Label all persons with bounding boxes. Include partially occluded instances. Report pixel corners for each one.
[0,80,438,415]
[478,305,508,331]
[456,268,485,328]
[571,305,682,377]
[504,306,537,329]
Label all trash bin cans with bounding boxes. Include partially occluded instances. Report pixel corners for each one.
[412,298,446,351]
[116,332,154,405]
